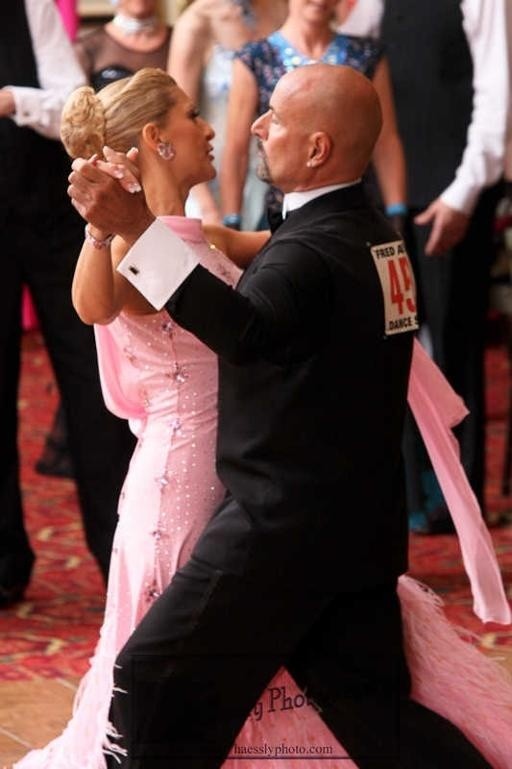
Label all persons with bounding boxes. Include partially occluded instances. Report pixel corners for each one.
[66,60,494,769]
[59,65,277,769]
[0,2,511,769]
